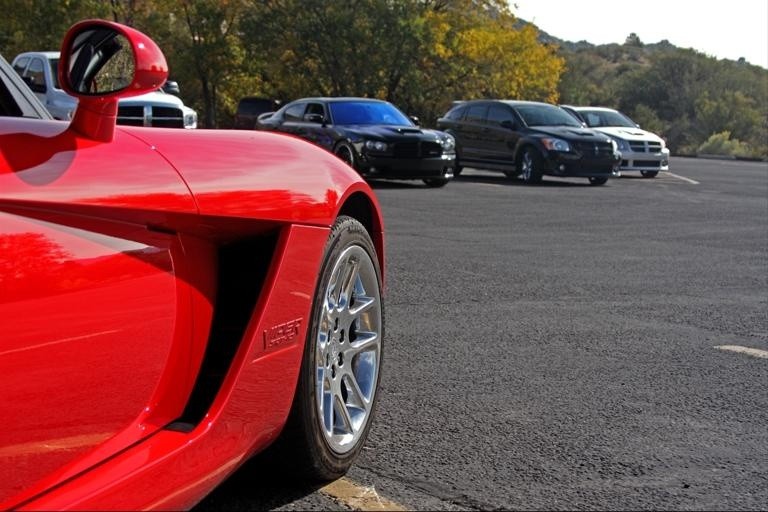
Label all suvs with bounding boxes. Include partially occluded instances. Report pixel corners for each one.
[12,44,200,129]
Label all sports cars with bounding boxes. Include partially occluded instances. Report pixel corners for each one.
[0,13,387,512]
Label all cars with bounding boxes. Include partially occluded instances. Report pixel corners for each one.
[562,104,671,180]
[253,94,464,189]
[434,99,625,189]
